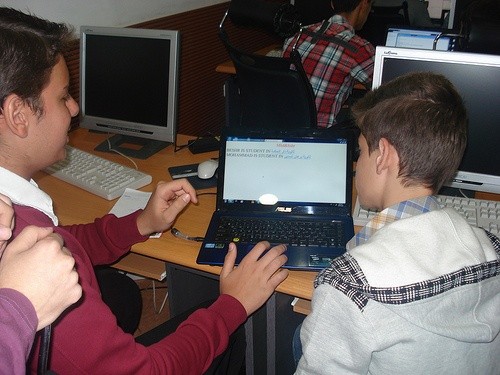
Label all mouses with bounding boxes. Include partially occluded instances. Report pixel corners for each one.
[197,158,219,179]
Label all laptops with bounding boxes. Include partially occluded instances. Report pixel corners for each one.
[195,127,355,272]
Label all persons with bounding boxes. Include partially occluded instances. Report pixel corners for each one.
[0,194,83,375]
[282,0,376,129]
[291,70,500,375]
[0,6,289,375]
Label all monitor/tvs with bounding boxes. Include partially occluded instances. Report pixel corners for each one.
[385,26,458,52]
[77,26,181,143]
[373,46,499,196]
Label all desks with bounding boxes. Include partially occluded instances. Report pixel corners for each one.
[267,178,369,375]
[215,43,369,127]
[30,119,267,375]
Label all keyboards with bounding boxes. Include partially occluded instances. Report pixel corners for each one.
[352,193,500,239]
[39,144,153,200]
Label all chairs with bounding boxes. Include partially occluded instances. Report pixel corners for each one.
[203,0,500,135]
